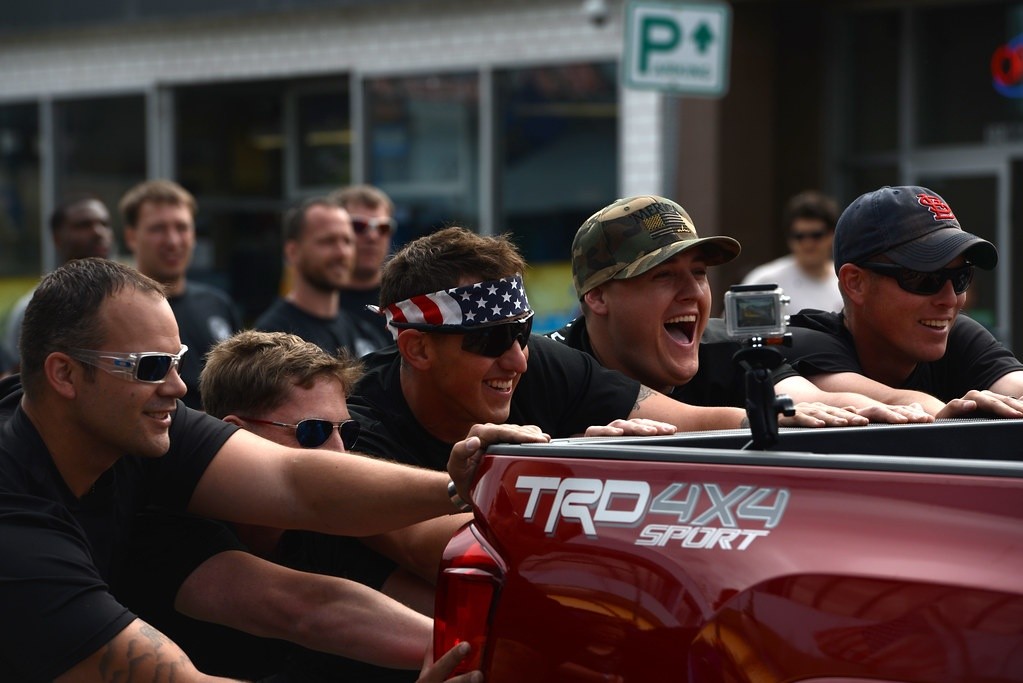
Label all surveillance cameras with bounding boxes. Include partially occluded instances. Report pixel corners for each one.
[583,0,608,26]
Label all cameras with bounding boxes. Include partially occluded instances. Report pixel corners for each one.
[724,283,791,339]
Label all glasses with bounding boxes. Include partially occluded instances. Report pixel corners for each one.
[389,309,535,358]
[789,230,831,241]
[38,343,189,384]
[853,257,977,295]
[349,214,397,237]
[237,414,361,450]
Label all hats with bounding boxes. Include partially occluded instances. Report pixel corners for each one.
[571,195,742,302]
[833,186,999,276]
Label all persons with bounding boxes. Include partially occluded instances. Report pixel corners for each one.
[0,180,396,365]
[0,258,551,683]
[344,186,1023,578]
[161,327,438,683]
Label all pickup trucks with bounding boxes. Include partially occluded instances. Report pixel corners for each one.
[428,413,1022,683]
